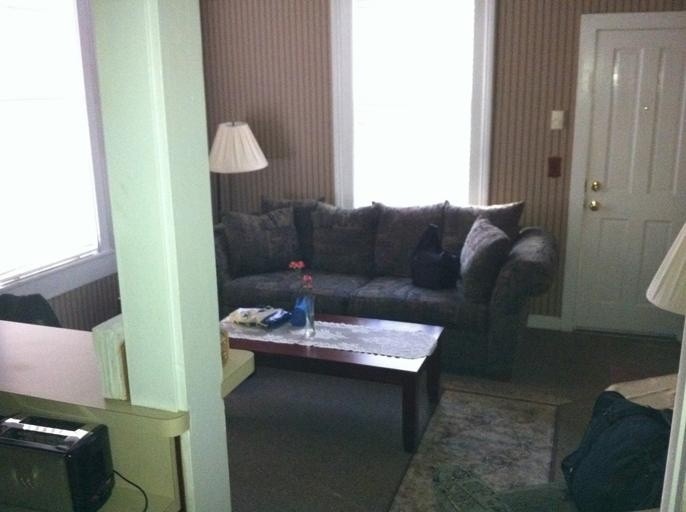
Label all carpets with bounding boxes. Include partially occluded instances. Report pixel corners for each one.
[384,382,562,512]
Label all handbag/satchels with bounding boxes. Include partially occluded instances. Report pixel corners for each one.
[411,224,455,289]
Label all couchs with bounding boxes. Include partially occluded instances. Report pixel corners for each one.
[211,193,558,385]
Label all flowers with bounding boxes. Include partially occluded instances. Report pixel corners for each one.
[288,250,315,301]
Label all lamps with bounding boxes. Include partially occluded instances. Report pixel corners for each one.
[207,116,271,216]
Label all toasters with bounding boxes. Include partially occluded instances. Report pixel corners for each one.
[0,414,115,511]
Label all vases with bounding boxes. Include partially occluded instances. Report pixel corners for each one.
[299,297,317,340]
